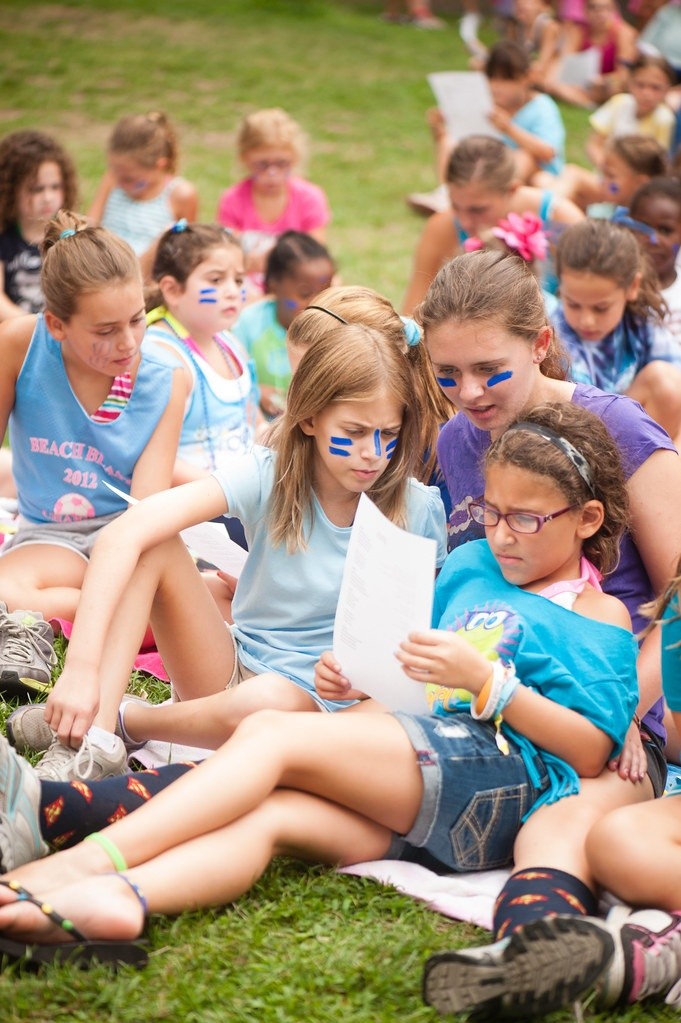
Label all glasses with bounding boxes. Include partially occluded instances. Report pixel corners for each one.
[467,494,586,534]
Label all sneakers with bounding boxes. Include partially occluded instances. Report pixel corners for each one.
[34,732,128,782]
[0,732,50,872]
[420,916,616,1014]
[605,905,681,1008]
[5,696,154,753]
[0,602,58,706]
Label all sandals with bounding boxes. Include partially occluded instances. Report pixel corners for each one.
[0,868,151,970]
[0,830,130,907]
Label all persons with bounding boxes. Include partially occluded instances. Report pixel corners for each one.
[383,0,681,110]
[0,45,681,1023]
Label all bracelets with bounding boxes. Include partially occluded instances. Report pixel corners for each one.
[470,661,520,721]
